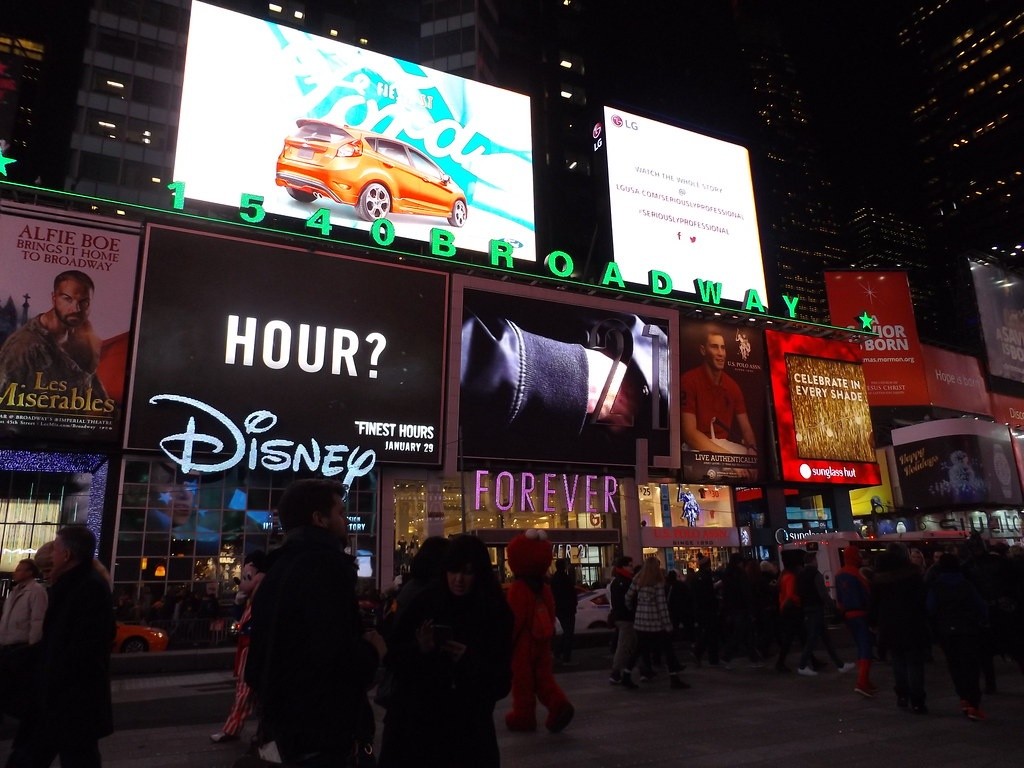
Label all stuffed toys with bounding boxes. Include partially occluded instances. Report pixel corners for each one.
[207,549,267,743]
[504,528,575,733]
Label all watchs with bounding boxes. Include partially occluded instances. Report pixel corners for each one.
[748,444,758,452]
[993,443,1012,498]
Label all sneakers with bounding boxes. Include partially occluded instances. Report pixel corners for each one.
[853,685,876,698]
[208,731,240,743]
[838,662,856,674]
[796,666,818,676]
[609,670,622,684]
[621,672,633,686]
[959,698,985,721]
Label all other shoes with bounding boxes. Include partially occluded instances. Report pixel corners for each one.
[670,674,681,689]
[912,702,928,714]
[897,697,909,708]
[638,676,657,690]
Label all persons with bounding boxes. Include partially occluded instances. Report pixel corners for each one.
[460,300,635,462]
[0,270,119,417]
[606,542,1024,721]
[550,559,578,656]
[118,460,195,541]
[680,324,758,456]
[244,479,516,768]
[0,526,116,768]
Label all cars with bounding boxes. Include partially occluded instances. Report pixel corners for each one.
[572,585,612,631]
[112,616,171,654]
[273,114,471,229]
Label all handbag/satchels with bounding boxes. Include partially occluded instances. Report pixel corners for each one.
[665,578,689,607]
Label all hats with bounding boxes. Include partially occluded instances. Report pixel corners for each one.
[695,552,712,567]
